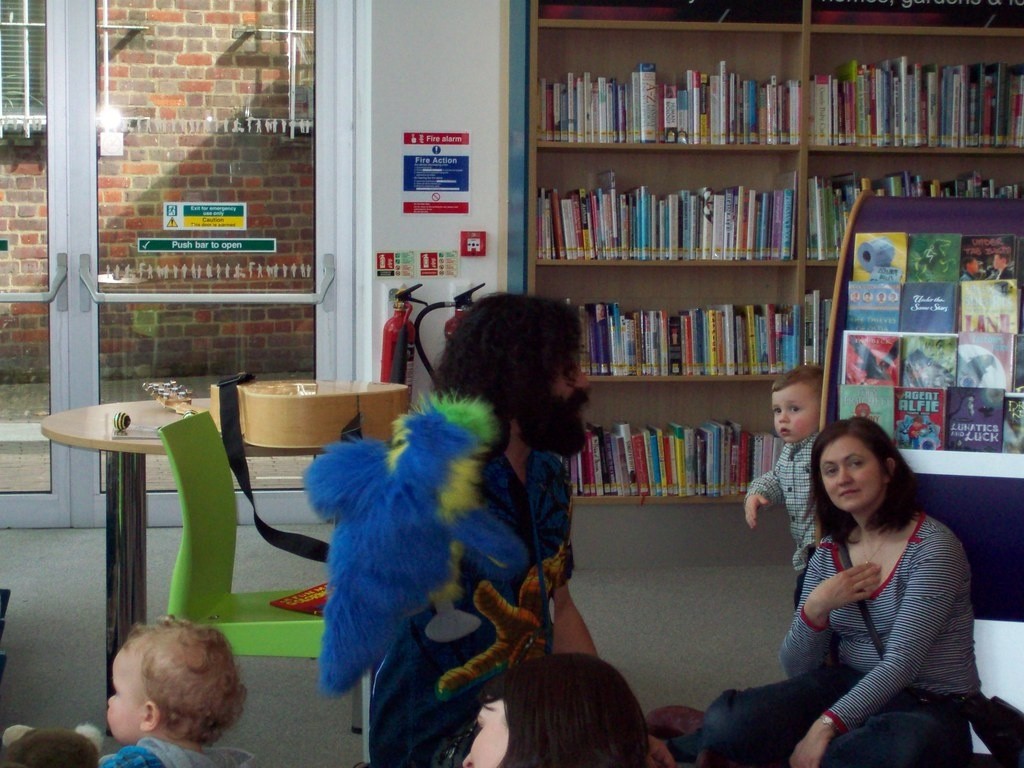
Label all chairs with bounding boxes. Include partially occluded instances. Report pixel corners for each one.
[157,411,382,765]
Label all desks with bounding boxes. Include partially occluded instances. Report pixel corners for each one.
[37,397,326,736]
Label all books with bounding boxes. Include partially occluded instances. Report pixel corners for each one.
[810,55,1024,149]
[271,581,332,616]
[539,60,802,145]
[537,169,798,260]
[565,298,801,376]
[804,168,1024,454]
[561,414,783,496]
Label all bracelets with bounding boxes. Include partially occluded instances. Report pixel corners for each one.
[820,716,840,735]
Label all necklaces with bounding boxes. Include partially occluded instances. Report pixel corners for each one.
[851,527,892,566]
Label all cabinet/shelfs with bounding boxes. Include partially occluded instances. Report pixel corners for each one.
[529,0,1024,508]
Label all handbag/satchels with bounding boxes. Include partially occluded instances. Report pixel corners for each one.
[963,693,1024,768]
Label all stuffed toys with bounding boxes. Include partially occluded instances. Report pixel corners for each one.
[0,723,103,768]
[304,392,528,702]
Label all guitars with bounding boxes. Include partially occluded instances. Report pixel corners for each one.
[140,374,411,449]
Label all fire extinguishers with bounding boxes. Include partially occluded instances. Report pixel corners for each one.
[382,284,430,407]
[414,282,487,386]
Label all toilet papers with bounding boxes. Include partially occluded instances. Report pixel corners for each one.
[858,237,896,271]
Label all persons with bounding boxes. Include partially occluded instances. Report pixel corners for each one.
[462,654,648,768]
[368,293,676,768]
[744,364,823,610]
[99,614,258,768]
[666,417,988,768]
